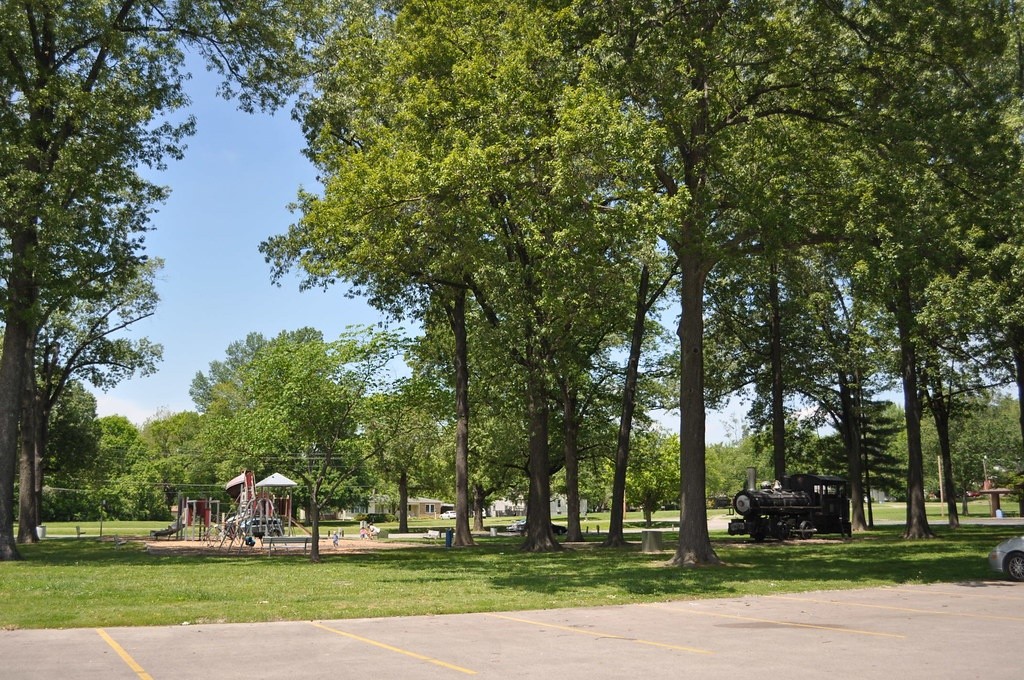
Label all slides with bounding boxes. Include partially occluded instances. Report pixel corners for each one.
[150,521,185,536]
[286,515,311,535]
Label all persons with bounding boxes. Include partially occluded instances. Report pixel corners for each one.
[332,532,339,549]
[245,536,255,555]
[360,522,380,540]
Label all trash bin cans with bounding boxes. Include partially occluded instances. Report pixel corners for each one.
[490,525,498,536]
[36,525,46,538]
[995,509,1002,518]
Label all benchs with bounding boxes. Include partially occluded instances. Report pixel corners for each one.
[423,529,440,546]
[76,525,86,538]
[261,536,312,559]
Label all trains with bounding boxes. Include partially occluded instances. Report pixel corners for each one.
[727,467,851,541]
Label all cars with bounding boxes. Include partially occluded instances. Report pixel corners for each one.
[987,535,1024,582]
[517,517,567,536]
[439,511,457,519]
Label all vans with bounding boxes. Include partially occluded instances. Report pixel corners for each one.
[241,516,284,538]
